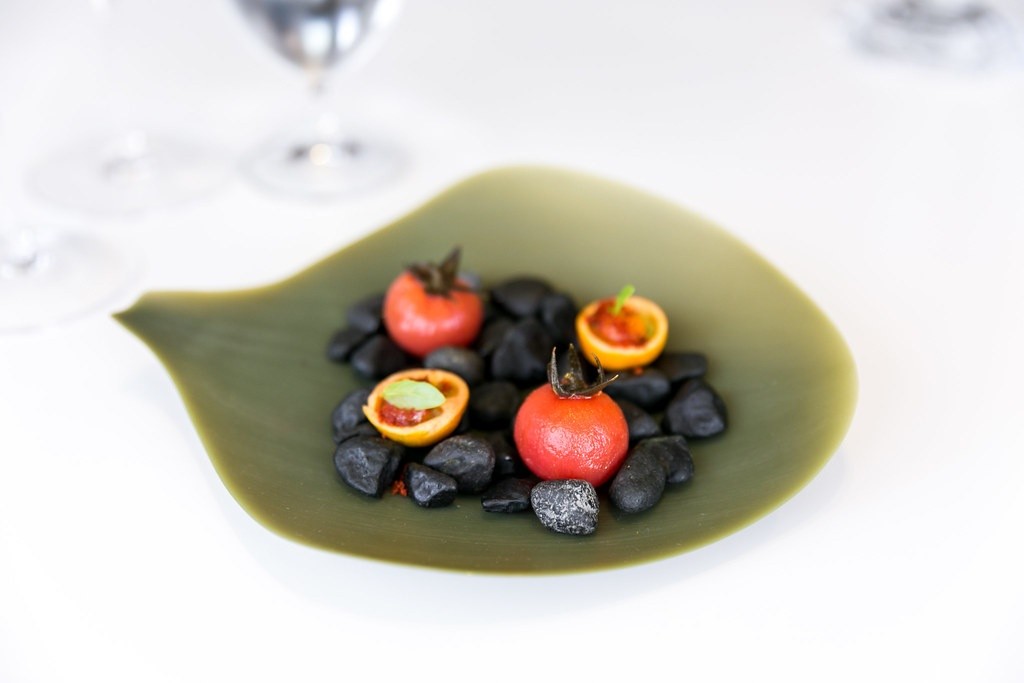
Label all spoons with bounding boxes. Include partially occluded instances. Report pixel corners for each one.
[230,0,375,70]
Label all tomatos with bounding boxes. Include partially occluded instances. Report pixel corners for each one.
[382,263,484,356]
[512,381,629,488]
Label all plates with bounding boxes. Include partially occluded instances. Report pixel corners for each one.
[109,162,860,576]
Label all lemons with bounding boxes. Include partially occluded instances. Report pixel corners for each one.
[363,369,469,447]
[574,294,669,372]
